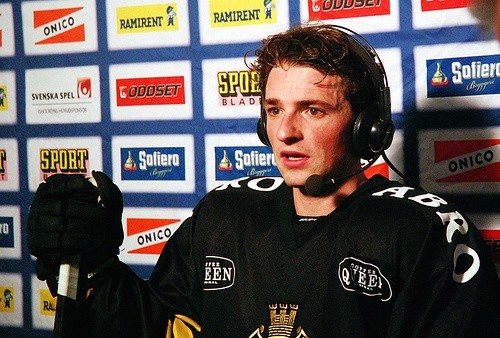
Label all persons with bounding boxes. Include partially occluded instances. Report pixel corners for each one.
[25,24,500,338]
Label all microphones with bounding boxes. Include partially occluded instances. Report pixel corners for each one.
[304,124,394,196]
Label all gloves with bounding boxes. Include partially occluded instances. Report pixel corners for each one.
[25,170,124,298]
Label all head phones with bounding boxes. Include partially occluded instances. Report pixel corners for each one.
[256,27,396,159]
